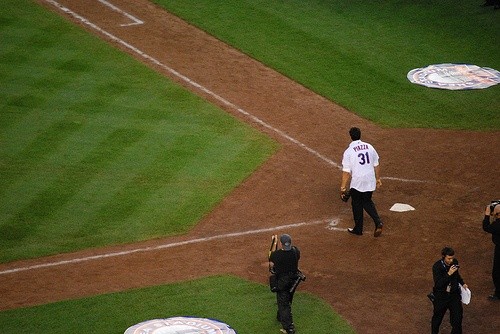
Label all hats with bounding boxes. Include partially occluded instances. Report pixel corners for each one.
[280,234,291,247]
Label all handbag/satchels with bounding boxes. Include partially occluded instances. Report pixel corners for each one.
[270,274,277,292]
[427,294,434,304]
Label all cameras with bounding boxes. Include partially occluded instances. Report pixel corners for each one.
[341,189,351,202]
[485,200,500,216]
[290,273,306,293]
[452,265,460,270]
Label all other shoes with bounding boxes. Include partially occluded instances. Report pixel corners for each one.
[488,294,499,300]
[348,229,362,234]
[374,221,383,237]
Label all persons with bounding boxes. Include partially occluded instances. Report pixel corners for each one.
[340,127,384,237]
[431,247,468,334]
[269,234,301,334]
[482,204,500,300]
